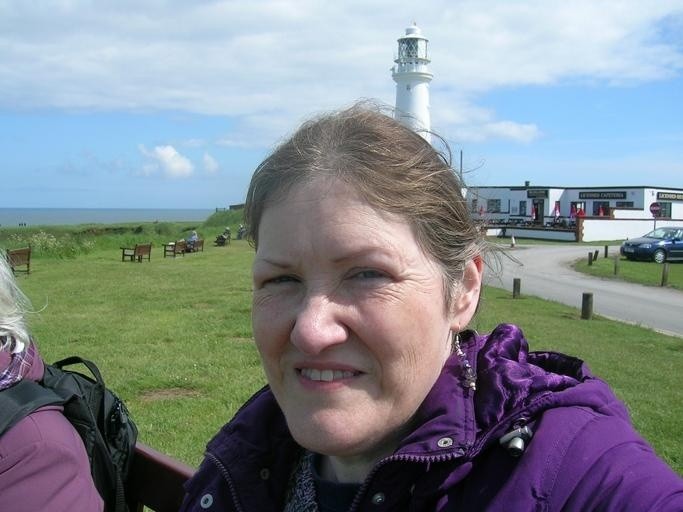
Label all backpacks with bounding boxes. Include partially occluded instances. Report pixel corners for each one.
[0,356,144,512]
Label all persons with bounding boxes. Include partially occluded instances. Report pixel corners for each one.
[213,227,230,245]
[0,237,108,512]
[187,231,197,245]
[179,98,683,512]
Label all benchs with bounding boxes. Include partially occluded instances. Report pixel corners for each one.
[186,239,203,253]
[216,233,231,246]
[487,218,561,228]
[119,242,151,263]
[568,220,576,228]
[6,243,29,273]
[161,240,186,258]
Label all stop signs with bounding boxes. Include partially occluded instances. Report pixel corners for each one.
[650,203,660,214]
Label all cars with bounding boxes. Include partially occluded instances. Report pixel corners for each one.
[620,226,683,264]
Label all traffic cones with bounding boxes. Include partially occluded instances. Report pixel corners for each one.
[510,233,517,249]
